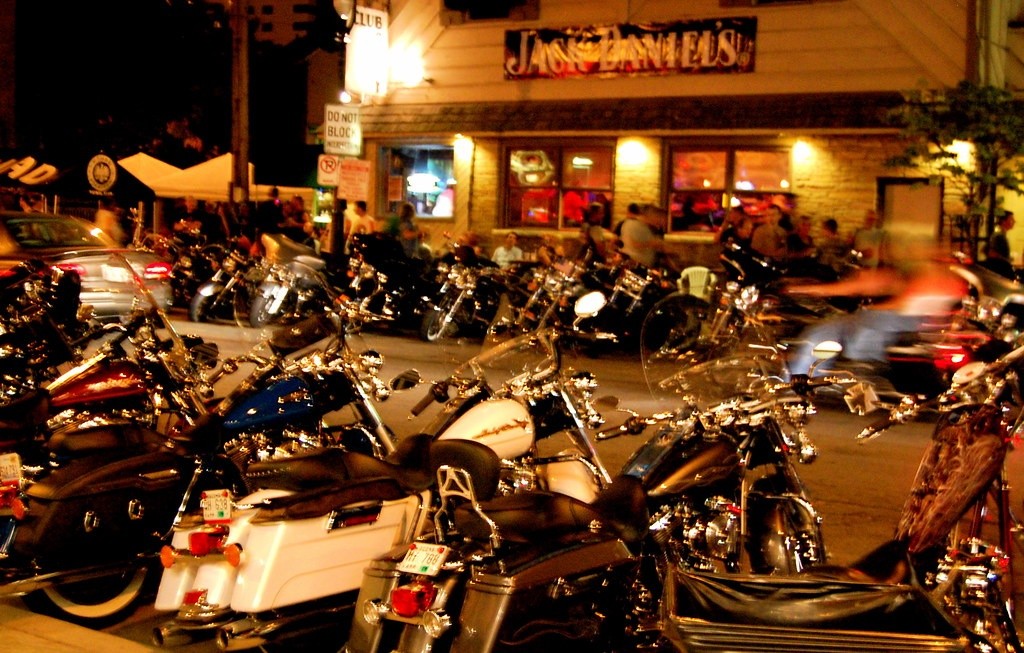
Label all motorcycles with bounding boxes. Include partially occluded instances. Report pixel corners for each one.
[1,213,1024,653]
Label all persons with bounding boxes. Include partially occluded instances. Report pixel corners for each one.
[397,202,429,259]
[580,193,893,271]
[981,209,1016,262]
[172,186,313,264]
[2,190,44,212]
[491,232,523,273]
[345,200,376,255]
[94,195,134,247]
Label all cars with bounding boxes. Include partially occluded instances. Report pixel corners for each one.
[775,251,1023,410]
[0,210,175,313]
